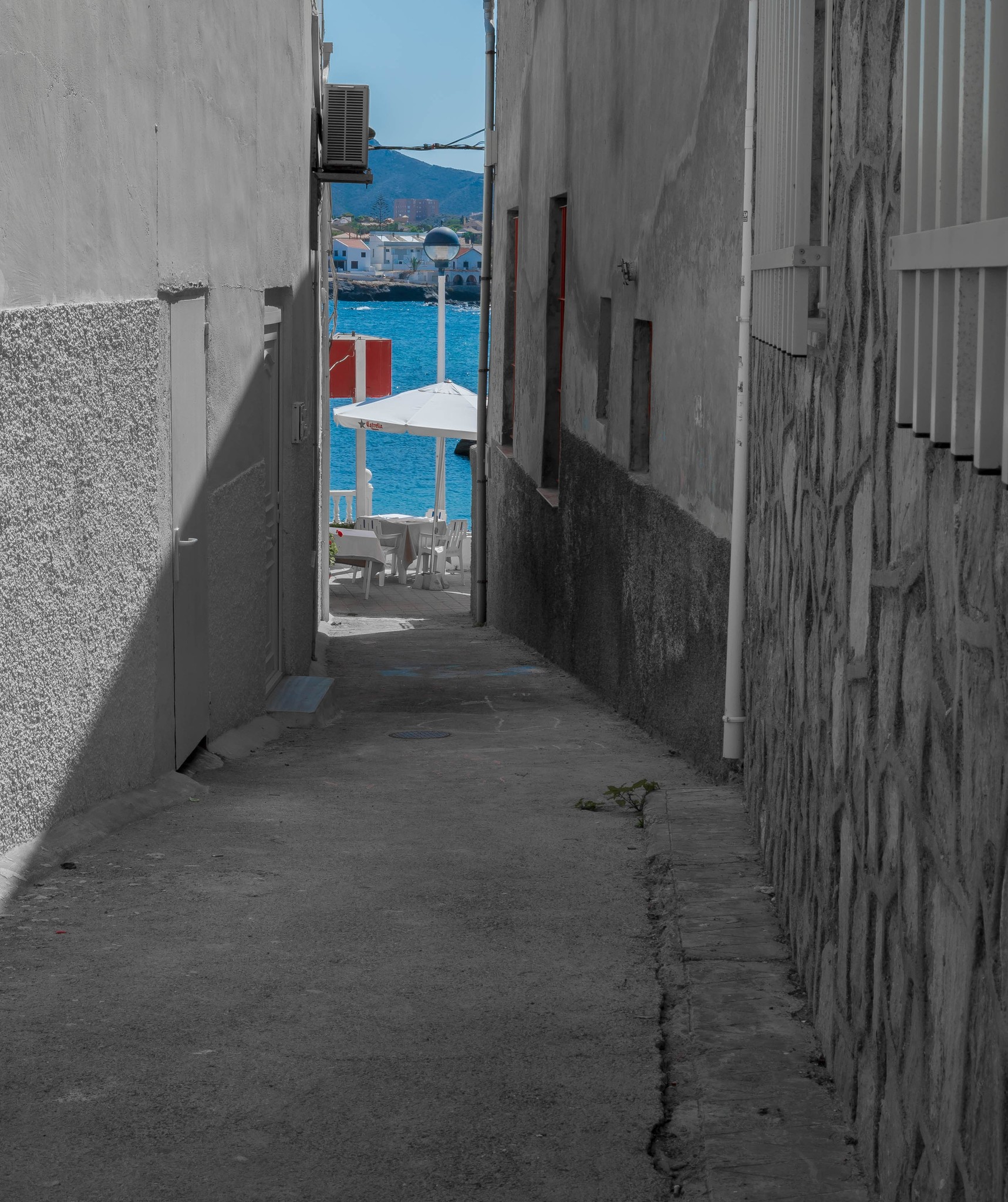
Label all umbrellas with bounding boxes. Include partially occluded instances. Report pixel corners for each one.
[332,375,489,576]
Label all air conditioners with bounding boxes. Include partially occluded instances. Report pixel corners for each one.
[321,83,371,172]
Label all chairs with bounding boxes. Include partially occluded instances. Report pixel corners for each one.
[421,509,447,572]
[356,516,402,583]
[415,519,467,582]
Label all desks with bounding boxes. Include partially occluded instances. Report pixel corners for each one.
[329,527,384,599]
[391,517,445,584]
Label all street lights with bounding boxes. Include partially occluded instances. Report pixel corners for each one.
[422,220,461,516]
